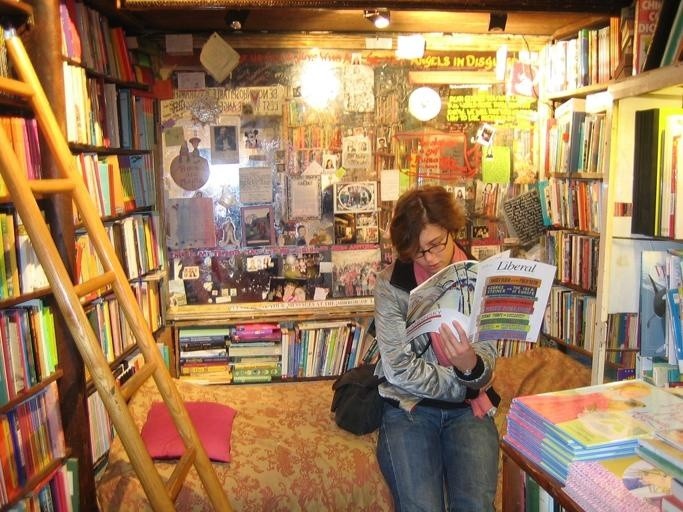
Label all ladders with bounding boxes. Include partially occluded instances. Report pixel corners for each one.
[0,36,232,512]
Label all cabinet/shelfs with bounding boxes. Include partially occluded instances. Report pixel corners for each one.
[0,1,175,511]
[164,295,378,388]
[544,0,683,407]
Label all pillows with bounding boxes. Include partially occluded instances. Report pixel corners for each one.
[490,347,608,414]
[141,399,237,463]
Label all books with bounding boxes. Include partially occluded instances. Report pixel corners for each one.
[2,1,683,512]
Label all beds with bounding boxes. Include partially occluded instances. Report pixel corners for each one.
[95,347,609,512]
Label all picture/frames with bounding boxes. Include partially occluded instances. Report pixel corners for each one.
[241,205,277,248]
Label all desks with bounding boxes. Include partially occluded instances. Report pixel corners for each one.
[498,440,589,511]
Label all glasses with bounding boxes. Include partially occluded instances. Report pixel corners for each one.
[413,228,449,259]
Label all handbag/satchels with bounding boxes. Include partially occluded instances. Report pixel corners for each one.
[331,362,384,434]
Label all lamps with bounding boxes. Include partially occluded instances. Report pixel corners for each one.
[486,142,494,160]
[364,8,390,30]
[223,9,249,31]
[488,11,507,33]
